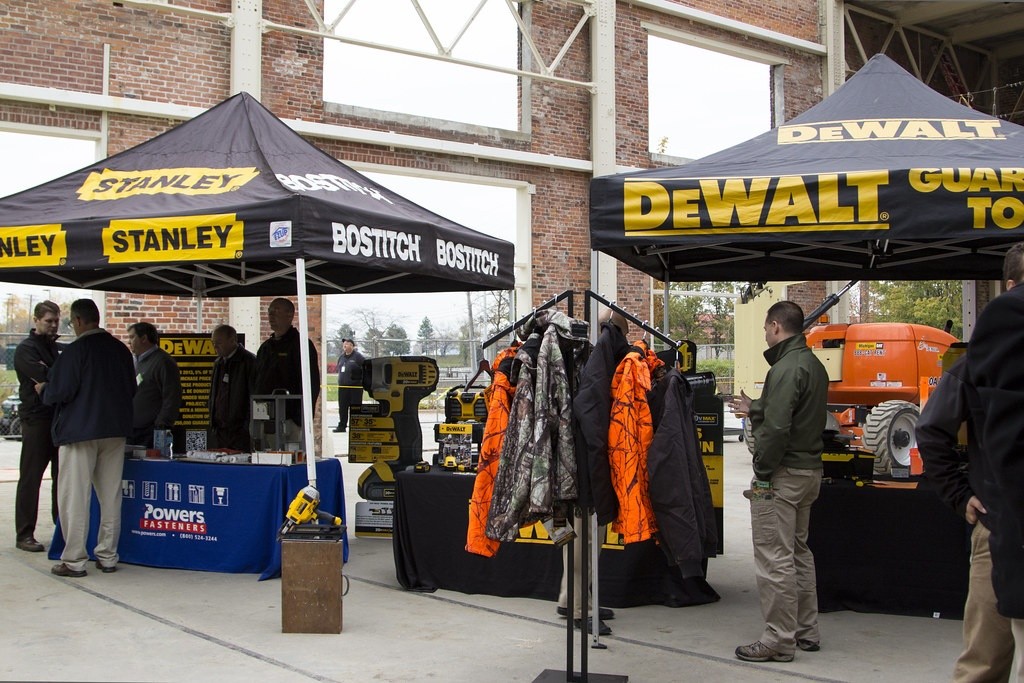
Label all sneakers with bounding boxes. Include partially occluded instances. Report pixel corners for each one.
[557,601,615,620]
[16,536,44,552]
[796,639,820,651]
[96,559,116,572]
[51,562,87,577]
[574,614,611,635]
[735,641,794,662]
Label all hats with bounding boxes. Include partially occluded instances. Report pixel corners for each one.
[341,337,355,347]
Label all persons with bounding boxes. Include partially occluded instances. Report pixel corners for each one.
[333,339,366,432]
[915,240,1024,683]
[206,297,321,455]
[727,300,830,662]
[15,300,184,576]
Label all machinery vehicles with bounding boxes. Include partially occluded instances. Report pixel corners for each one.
[799,280,968,621]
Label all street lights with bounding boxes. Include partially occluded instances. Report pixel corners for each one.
[44,289,51,301]
[6,292,13,334]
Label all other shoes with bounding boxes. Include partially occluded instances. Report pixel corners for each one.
[332,427,346,432]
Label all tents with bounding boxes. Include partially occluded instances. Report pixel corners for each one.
[589,54,1024,650]
[0,91,517,539]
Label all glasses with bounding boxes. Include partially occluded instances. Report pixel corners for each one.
[67,317,81,328]
